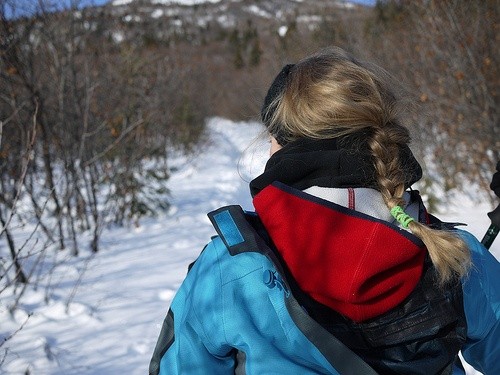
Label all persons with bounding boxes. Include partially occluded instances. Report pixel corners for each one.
[148,46,500,375]
